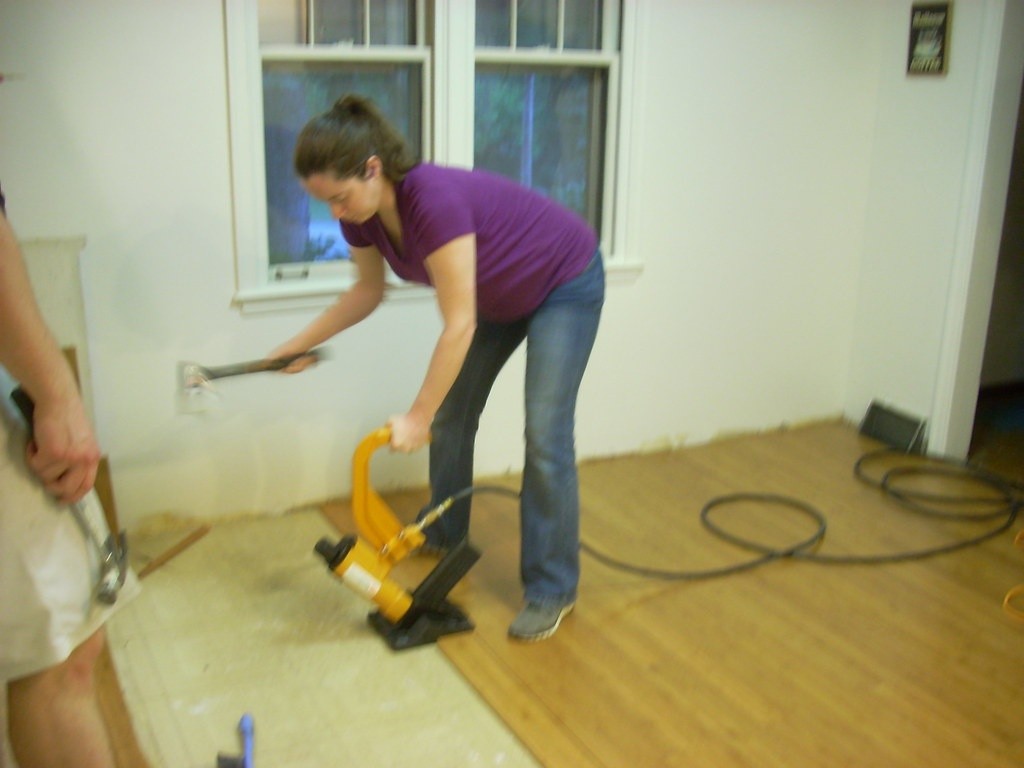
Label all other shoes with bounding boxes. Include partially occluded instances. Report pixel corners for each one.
[414,511,467,554]
[507,599,576,642]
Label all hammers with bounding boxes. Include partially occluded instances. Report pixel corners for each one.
[11,387,129,605]
[173,349,327,415]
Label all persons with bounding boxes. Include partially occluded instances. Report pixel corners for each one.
[0,187,116,768]
[266,94,606,639]
